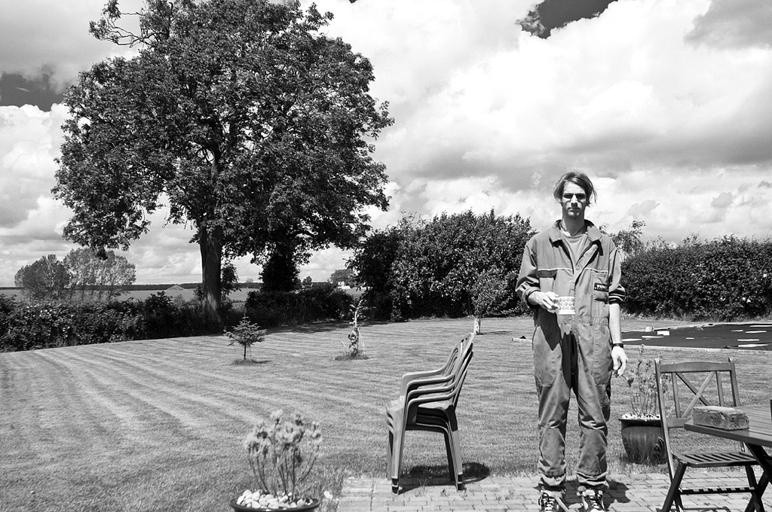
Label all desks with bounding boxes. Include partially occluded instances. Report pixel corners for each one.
[684,399,772,484]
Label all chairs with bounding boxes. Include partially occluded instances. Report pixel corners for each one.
[655,356,765,512]
[384,332,474,498]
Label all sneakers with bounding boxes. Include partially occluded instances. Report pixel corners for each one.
[583,488,604,511]
[539,489,566,512]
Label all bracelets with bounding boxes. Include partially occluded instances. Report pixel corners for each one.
[613,343,624,348]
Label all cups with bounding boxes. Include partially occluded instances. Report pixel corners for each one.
[555,296,578,316]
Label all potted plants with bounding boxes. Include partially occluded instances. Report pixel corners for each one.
[229,409,322,512]
[620,344,669,465]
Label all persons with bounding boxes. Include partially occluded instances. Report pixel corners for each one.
[515,171,629,510]
